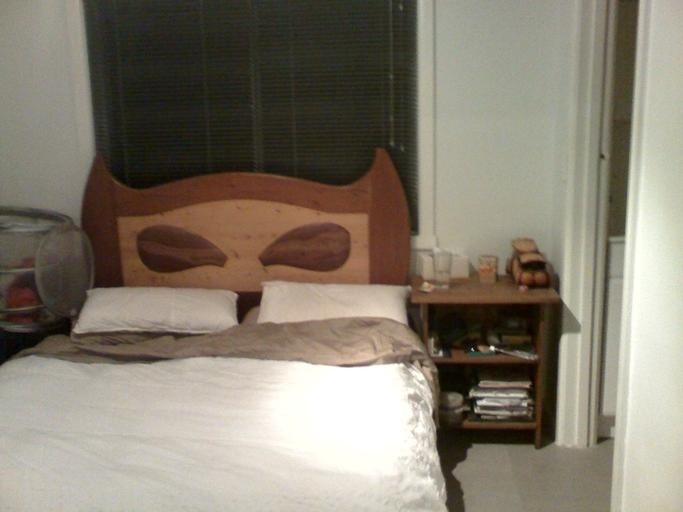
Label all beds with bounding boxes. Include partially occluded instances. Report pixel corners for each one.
[0,147,449,511]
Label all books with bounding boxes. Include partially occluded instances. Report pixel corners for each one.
[466,366,538,422]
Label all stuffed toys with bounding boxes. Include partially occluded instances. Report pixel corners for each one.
[503,235,557,289]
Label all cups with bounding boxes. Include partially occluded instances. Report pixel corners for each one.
[431,246,452,291]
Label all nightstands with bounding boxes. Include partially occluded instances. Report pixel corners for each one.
[408,273,561,449]
